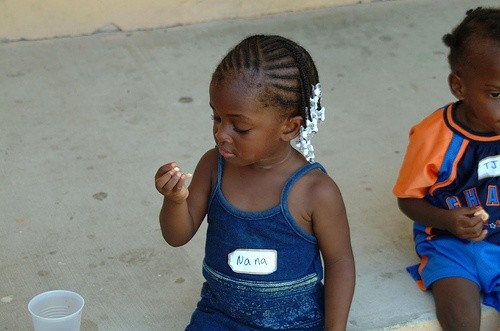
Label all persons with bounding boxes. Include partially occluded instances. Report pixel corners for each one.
[392,8,500,331]
[155,33,355,331]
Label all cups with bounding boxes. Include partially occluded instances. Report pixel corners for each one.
[28,290,84,331]
